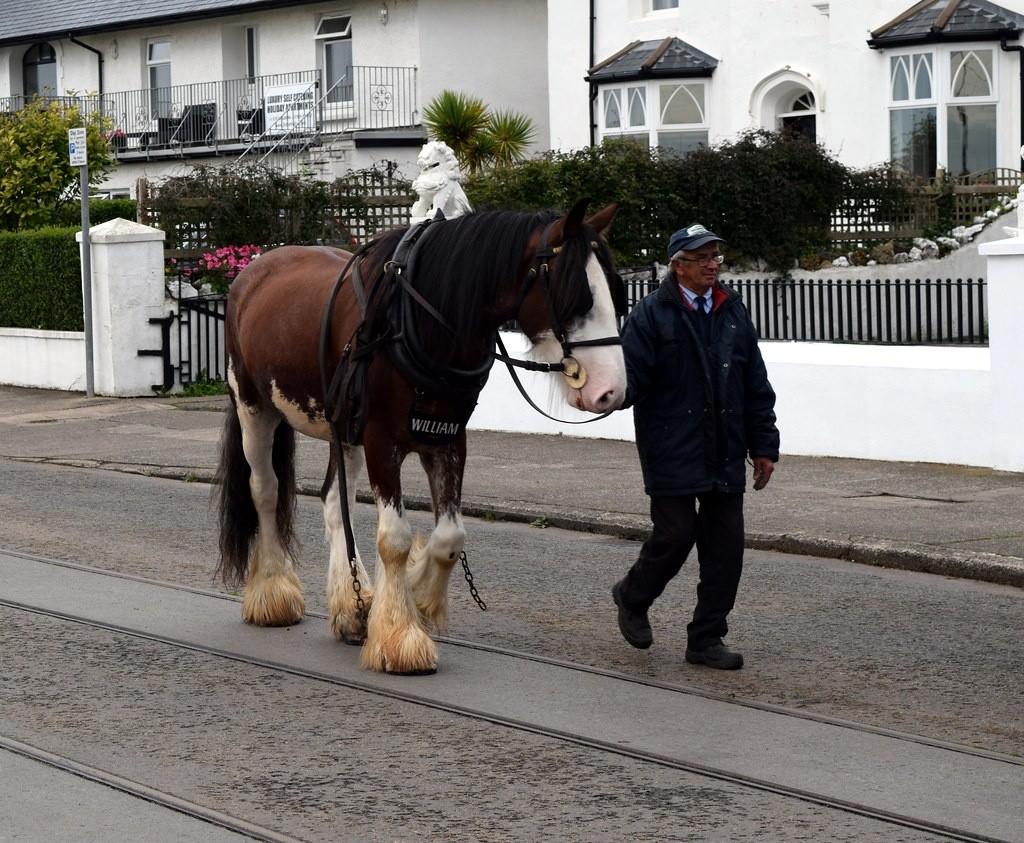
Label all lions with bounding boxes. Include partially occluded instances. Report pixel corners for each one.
[411,139,473,217]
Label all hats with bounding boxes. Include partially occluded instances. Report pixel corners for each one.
[668,223,727,257]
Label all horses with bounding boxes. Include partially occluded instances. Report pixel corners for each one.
[206,196,630,678]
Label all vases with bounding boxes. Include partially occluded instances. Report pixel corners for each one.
[111,135,126,153]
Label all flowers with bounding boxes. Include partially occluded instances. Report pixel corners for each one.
[100,128,125,140]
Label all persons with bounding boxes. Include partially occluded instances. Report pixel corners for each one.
[611,224,780,671]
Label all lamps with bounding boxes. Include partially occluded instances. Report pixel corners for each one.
[110,39,118,60]
[377,2,388,26]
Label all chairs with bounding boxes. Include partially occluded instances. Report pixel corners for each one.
[237,109,265,136]
[158,103,216,144]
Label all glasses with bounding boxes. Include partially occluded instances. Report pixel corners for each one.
[676,255,724,267]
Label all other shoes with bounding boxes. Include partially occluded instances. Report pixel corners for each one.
[685,644,743,669]
[612,581,652,649]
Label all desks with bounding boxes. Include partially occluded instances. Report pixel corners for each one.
[127,132,158,151]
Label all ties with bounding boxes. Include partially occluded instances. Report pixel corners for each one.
[694,296,706,314]
